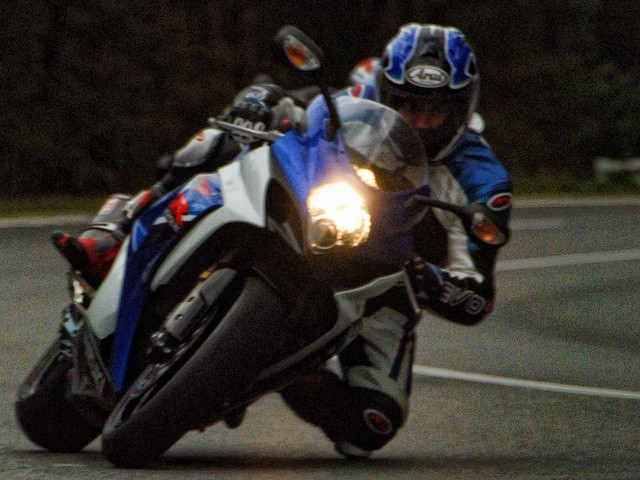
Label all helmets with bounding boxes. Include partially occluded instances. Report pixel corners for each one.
[375,20,480,163]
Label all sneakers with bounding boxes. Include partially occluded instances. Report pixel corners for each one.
[51,230,120,289]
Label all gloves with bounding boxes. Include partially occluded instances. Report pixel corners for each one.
[222,80,298,147]
[386,252,447,317]
